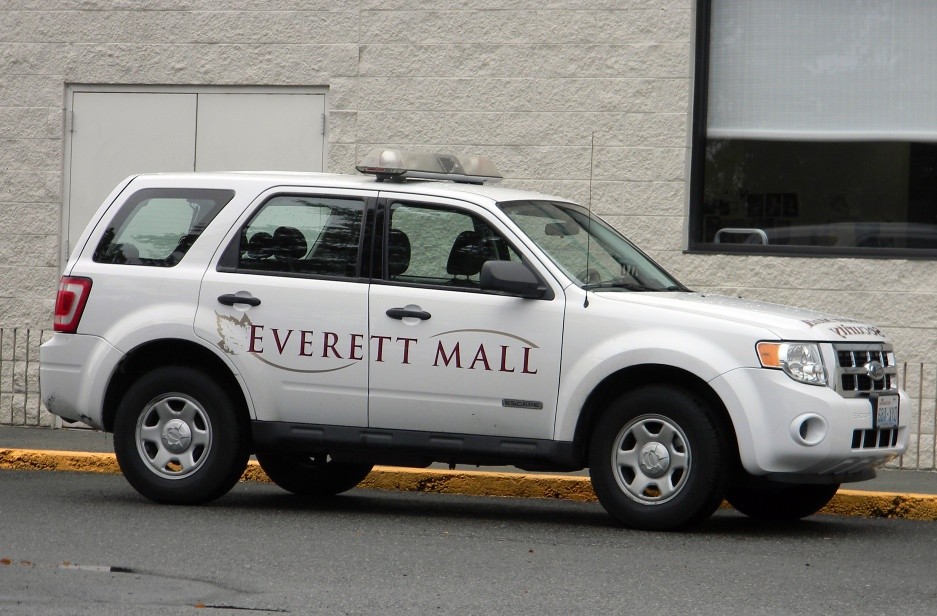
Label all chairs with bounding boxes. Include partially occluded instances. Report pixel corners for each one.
[391,229,412,278]
[445,230,491,287]
[273,227,307,259]
[248,233,272,259]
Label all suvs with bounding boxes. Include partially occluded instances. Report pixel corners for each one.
[40,147,911,527]
